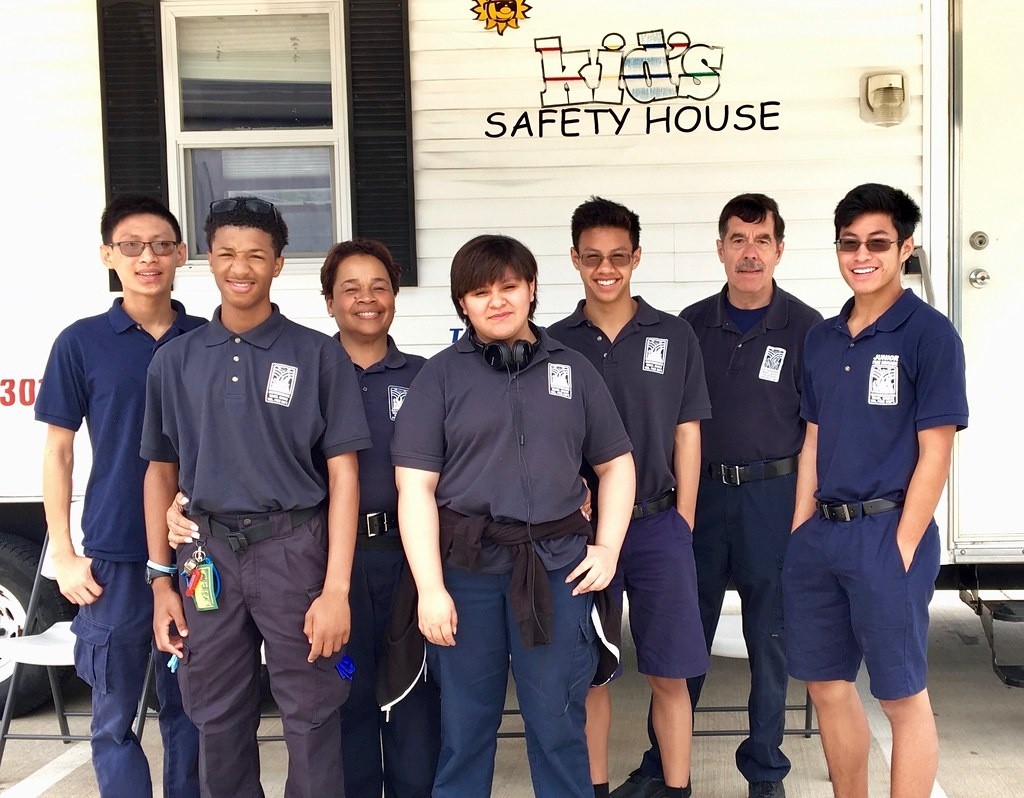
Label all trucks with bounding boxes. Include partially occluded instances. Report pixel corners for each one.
[0,0,1024,720]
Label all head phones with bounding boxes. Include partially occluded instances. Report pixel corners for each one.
[466,319,542,370]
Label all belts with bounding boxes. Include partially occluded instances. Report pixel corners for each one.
[700,452,802,488]
[631,491,679,518]
[354,508,398,537]
[815,492,907,522]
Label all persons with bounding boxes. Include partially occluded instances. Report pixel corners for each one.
[390,235,637,798]
[33,193,209,798]
[543,197,713,798]
[166,237,441,798]
[138,198,372,798]
[606,193,823,798]
[780,183,969,798]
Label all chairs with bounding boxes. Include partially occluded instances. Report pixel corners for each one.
[1,525,831,779]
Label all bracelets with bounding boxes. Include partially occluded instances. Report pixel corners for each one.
[146,559,177,573]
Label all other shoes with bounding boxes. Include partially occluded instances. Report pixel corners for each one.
[653,776,692,798]
[608,768,665,798]
[747,781,784,798]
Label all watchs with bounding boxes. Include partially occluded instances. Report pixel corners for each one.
[145,566,173,584]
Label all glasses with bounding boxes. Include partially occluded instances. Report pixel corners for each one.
[108,240,179,257]
[833,235,910,252]
[577,252,635,267]
[209,198,278,224]
